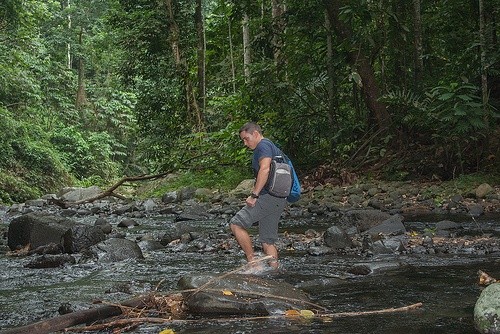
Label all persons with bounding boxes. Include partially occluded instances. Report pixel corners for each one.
[229,123,293,271]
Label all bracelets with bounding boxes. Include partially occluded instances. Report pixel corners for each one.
[249,194,259,200]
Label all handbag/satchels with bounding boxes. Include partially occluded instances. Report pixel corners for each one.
[279,150,301,203]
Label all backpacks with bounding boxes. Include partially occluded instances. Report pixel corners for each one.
[261,138,294,198]
